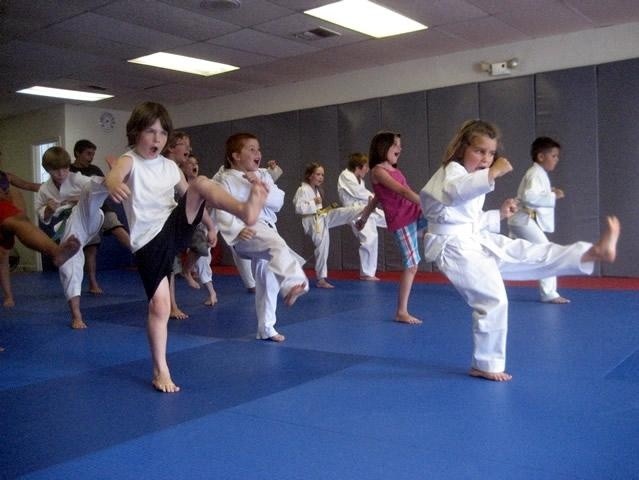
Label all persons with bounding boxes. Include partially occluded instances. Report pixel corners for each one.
[355,129,428,324]
[106,104,270,394]
[420,118,621,381]
[0,139,131,329]
[291,162,373,289]
[336,152,389,281]
[162,131,311,342]
[506,136,572,305]
